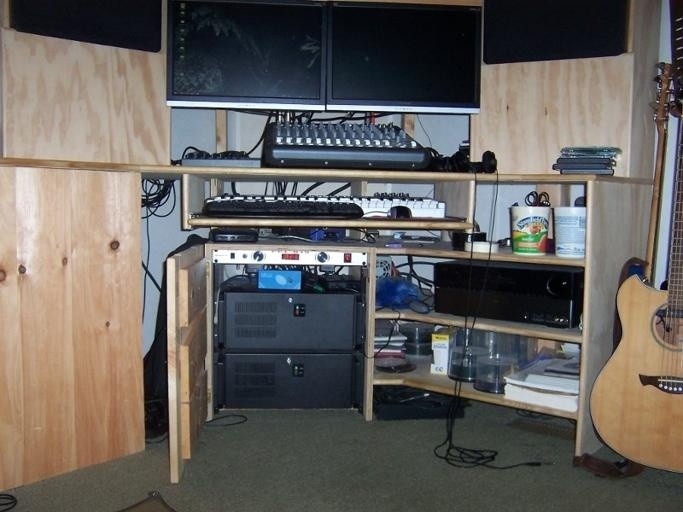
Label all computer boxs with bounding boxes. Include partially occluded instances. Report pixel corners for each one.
[213,351,365,409]
[213,278,366,351]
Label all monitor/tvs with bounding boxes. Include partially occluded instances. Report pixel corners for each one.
[483,0,631,65]
[166,0,327,111]
[326,0,483,114]
[9,0,163,53]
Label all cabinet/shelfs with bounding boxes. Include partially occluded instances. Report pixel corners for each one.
[142,168,656,485]
[0,0,172,170]
[468,0,662,181]
[0,159,145,490]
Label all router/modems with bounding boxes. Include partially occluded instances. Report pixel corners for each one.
[209,229,258,243]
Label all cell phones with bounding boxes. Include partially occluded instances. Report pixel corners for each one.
[403,236,437,244]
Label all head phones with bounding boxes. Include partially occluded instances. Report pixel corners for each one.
[449,150,497,174]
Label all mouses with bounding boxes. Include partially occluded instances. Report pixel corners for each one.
[387,205,413,221]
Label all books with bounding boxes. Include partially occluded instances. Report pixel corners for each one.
[374,332,408,358]
[503,346,581,413]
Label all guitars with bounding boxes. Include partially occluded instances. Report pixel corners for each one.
[589,1,683,474]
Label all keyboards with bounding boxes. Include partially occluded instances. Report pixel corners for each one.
[202,200,365,220]
